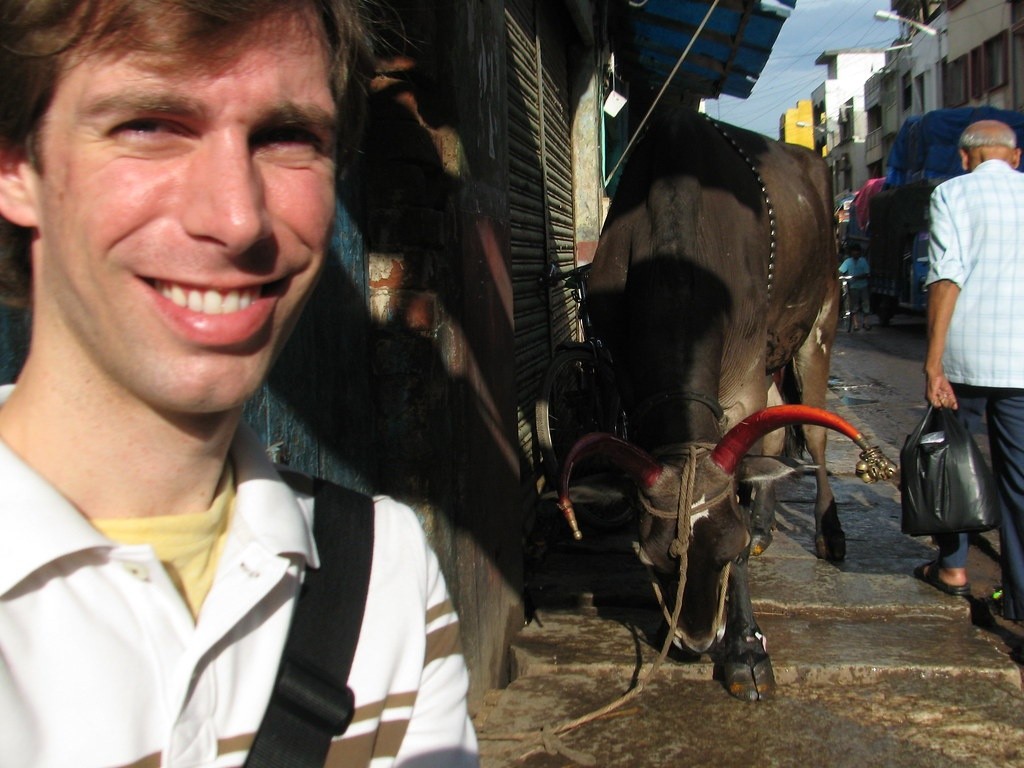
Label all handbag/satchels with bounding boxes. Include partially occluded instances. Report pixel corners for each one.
[900,409,1002,537]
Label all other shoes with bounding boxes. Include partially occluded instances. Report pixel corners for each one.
[855,328,860,330]
[863,324,872,330]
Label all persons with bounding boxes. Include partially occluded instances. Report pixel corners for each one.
[2,1,482,766]
[915,120,1023,624]
[838,244,871,331]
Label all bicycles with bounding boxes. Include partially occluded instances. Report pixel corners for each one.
[535,261,643,529]
[842,276,855,333]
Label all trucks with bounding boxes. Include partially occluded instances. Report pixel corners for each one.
[845,181,930,327]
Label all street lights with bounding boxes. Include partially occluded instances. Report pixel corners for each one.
[873,9,942,109]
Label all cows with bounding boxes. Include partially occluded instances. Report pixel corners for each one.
[560,114,870,701]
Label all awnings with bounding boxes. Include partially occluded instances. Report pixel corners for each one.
[619,0,797,99]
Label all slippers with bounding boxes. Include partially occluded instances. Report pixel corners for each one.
[913,562,971,595]
